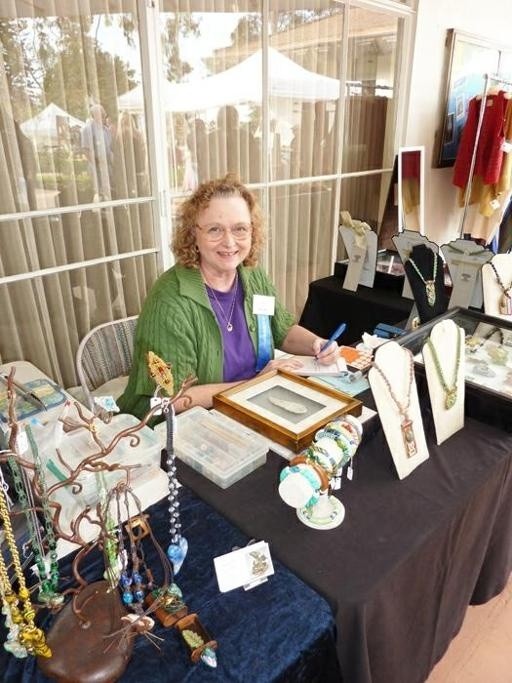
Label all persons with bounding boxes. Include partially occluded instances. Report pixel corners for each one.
[77,104,262,190]
[284,125,301,177]
[116,171,336,429]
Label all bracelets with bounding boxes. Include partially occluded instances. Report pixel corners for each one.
[279,416,359,513]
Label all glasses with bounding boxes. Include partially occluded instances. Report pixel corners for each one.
[194,221,255,242]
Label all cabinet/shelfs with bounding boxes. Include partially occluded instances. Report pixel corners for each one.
[372,304,510,428]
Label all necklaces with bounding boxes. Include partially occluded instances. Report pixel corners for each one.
[93,463,144,616]
[1,425,65,658]
[448,244,486,254]
[372,347,418,458]
[410,254,438,307]
[199,271,238,332]
[125,489,184,615]
[426,328,462,409]
[488,261,512,316]
[161,401,188,576]
[465,327,505,357]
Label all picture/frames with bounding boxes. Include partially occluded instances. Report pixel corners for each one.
[211,367,364,454]
[436,27,503,167]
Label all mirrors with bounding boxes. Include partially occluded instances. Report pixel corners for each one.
[397,145,425,236]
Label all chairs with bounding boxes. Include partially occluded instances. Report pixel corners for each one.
[77,314,138,416]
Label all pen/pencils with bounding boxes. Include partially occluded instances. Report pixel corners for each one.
[314,322,346,362]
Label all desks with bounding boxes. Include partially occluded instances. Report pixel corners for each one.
[1,359,334,682]
[155,398,512,683]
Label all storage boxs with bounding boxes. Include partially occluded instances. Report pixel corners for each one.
[154,405,268,488]
